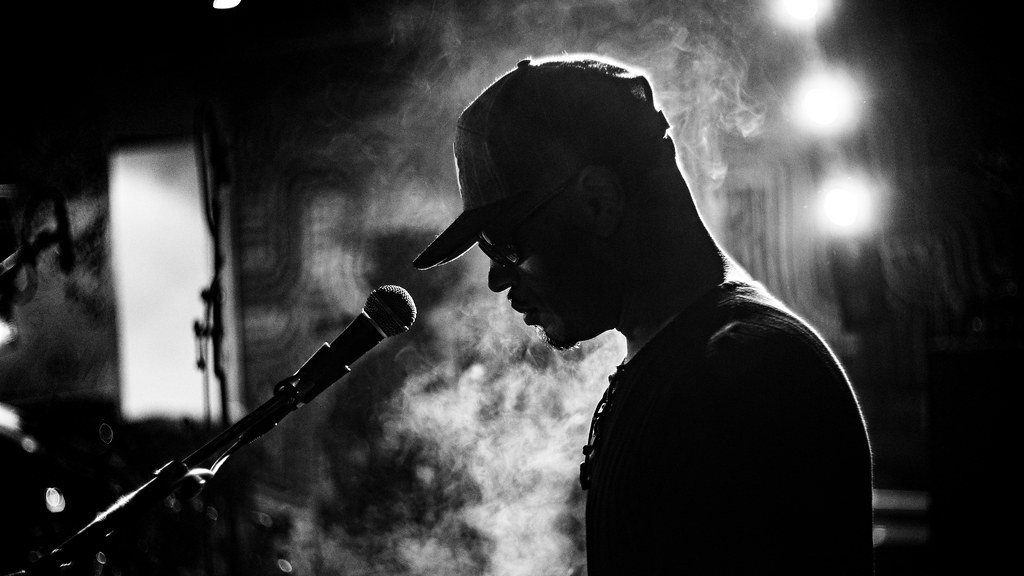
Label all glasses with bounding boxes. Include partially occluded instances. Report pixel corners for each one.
[478,155,623,267]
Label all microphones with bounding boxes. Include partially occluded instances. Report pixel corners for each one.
[228,284,417,453]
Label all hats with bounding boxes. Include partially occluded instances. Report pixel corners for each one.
[414,58,669,270]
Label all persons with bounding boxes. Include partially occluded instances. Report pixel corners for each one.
[412,53,875,576]
